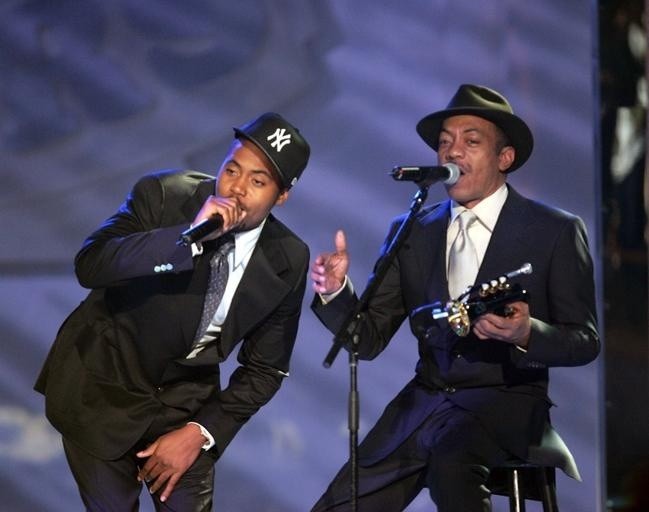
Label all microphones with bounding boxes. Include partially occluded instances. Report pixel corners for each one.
[175,213,223,249]
[387,162,461,186]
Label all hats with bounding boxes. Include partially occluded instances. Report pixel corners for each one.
[230,109,313,193]
[413,80,537,175]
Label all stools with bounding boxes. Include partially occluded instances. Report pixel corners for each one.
[492,427,560,512]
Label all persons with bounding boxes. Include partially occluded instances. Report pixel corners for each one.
[32,110,309,512]
[308,83,603,512]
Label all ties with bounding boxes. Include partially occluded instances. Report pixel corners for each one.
[446,208,482,304]
[190,237,236,357]
